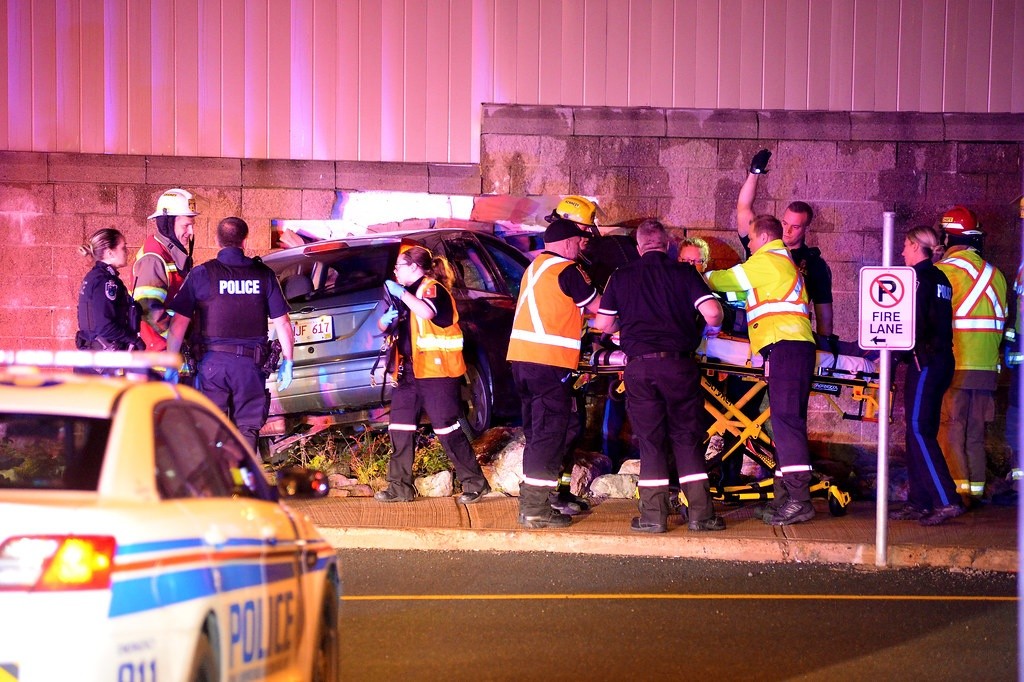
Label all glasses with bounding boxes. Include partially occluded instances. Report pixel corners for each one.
[680,255,704,265]
[395,263,411,271]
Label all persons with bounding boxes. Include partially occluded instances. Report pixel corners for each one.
[373,246,492,505]
[933,204,1009,507]
[128,187,200,382]
[164,216,294,455]
[889,224,967,528]
[74,227,148,375]
[702,213,818,526]
[504,148,834,533]
[593,219,724,533]
[992,194,1024,507]
[504,217,602,529]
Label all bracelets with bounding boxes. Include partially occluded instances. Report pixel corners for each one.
[402,290,406,294]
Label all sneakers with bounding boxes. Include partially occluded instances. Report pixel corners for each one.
[561,484,590,511]
[631,517,665,533]
[753,506,776,519]
[375,490,409,502]
[460,480,492,503]
[551,491,580,516]
[889,504,927,520]
[921,496,966,526]
[518,509,573,528]
[762,499,816,526]
[688,514,727,531]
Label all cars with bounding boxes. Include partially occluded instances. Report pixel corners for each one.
[258,228,531,440]
[0,348,342,682]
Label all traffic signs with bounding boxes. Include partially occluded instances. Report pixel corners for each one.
[858,266,916,350]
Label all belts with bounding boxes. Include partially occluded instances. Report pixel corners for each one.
[630,352,689,359]
[205,344,253,357]
[404,355,413,364]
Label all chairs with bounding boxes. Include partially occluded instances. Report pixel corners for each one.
[335,263,374,295]
[279,273,314,301]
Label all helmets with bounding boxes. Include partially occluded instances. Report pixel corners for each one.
[146,189,201,220]
[551,195,596,225]
[939,206,985,237]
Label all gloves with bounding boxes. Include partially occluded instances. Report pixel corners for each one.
[750,148,772,174]
[276,361,294,391]
[385,280,406,299]
[164,367,178,383]
[380,305,398,326]
